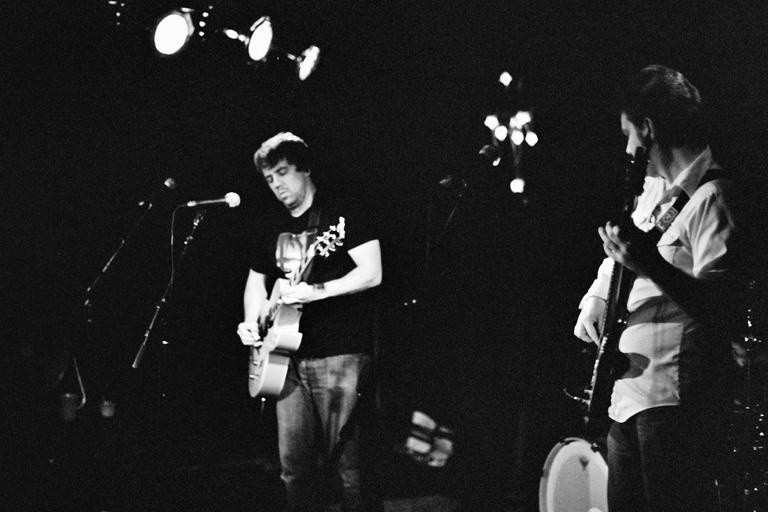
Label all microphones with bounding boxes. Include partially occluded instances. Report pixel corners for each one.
[147,177,175,211]
[437,145,497,188]
[187,192,241,208]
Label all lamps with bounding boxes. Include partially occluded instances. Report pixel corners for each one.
[126,1,325,86]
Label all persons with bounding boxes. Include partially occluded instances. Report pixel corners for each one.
[236,131,383,512]
[573,65,743,512]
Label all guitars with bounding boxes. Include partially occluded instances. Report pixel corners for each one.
[247,217,346,397]
[581,146,649,430]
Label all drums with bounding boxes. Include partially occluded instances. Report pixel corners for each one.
[536,435,607,512]
